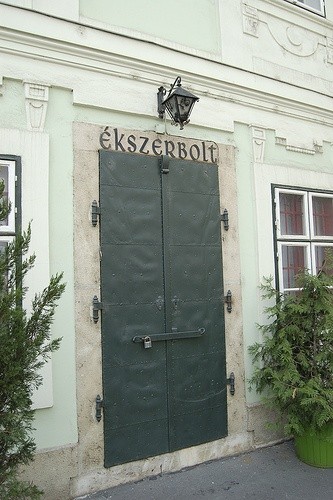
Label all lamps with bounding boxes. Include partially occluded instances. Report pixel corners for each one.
[157,76,200,130]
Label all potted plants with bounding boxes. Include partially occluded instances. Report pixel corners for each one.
[241,244,333,469]
